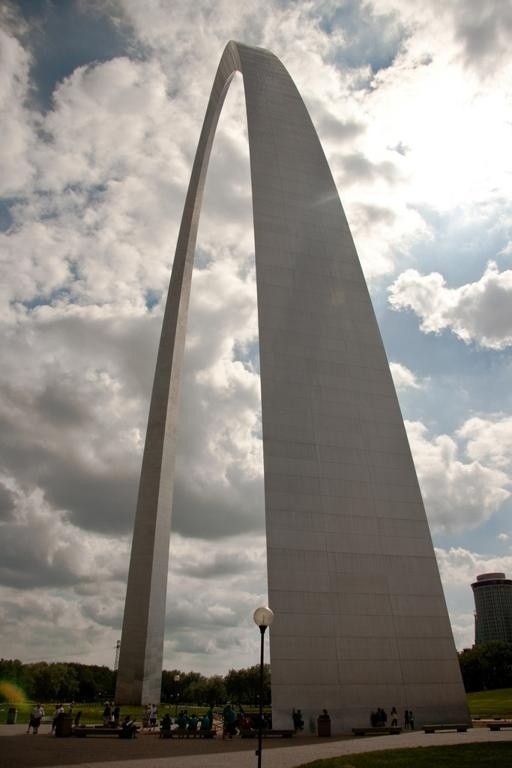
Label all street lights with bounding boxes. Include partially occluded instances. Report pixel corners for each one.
[253,607,274,768]
[174,674,181,716]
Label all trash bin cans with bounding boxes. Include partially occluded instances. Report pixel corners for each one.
[317,709,331,738]
[55,713,73,737]
[6,707,18,724]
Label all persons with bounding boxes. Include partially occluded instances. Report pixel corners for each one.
[122,716,140,739]
[223,705,272,739]
[292,708,304,734]
[371,708,387,727]
[27,704,45,734]
[405,710,414,730]
[102,701,120,728]
[48,705,64,735]
[391,707,397,726]
[141,703,214,738]
